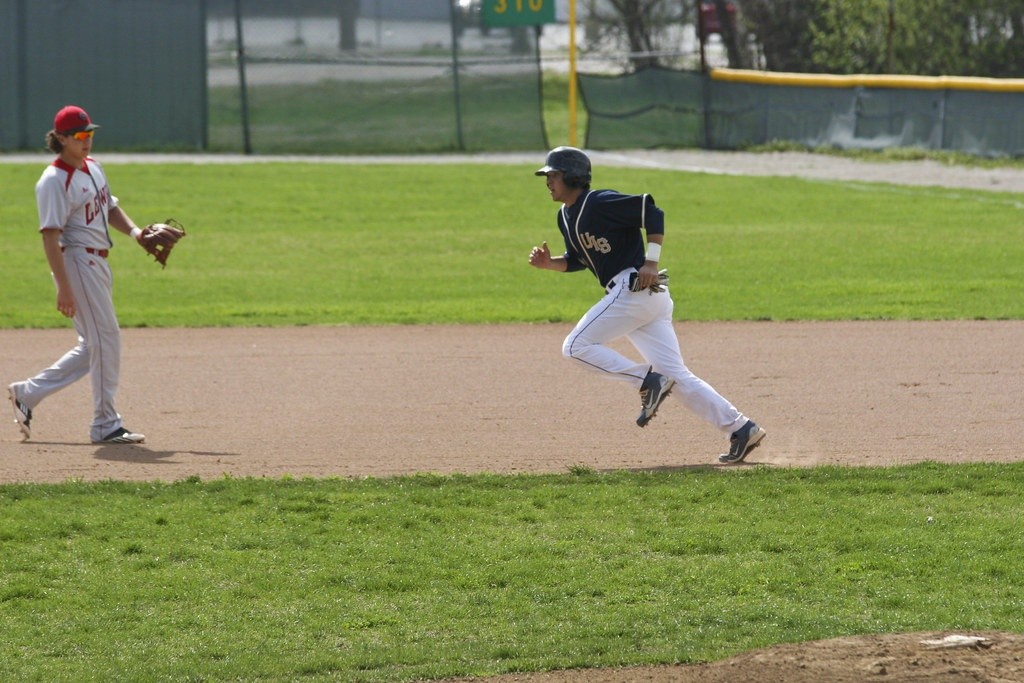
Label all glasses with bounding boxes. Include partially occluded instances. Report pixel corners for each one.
[74,132,95,141]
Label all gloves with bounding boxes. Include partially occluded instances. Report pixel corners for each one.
[629,269,669,293]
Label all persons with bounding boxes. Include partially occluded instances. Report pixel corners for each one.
[9,106,186,445]
[525,147,766,463]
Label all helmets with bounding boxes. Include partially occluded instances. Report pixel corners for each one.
[536,147,592,188]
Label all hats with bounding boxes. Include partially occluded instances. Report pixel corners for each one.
[54,106,100,135]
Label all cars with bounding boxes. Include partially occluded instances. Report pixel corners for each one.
[696,0,742,41]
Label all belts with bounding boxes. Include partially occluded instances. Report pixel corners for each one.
[605,281,616,295]
[60,248,108,257]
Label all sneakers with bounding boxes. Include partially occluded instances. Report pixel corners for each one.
[719,419,766,463]
[100,426,145,443]
[8,384,32,438]
[636,372,674,428]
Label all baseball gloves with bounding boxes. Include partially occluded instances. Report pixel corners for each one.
[136,217,187,270]
